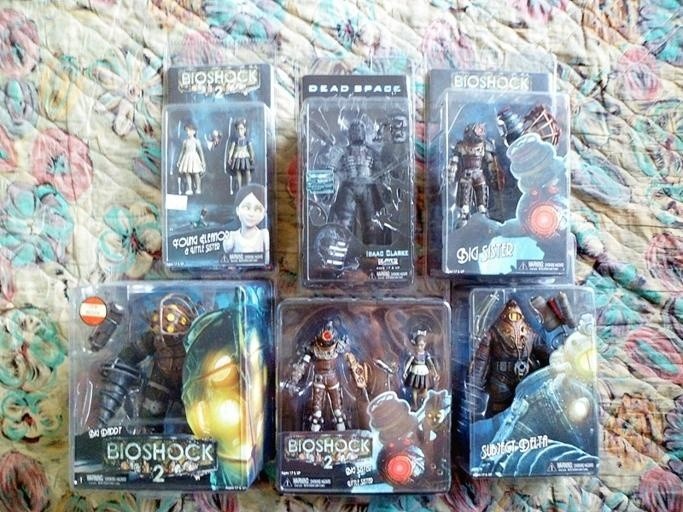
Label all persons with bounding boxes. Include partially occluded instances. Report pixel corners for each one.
[94,104,575,440]
[336,121,385,245]
[176,122,206,195]
[228,120,254,189]
[403,329,437,410]
[448,124,497,225]
[291,322,367,432]
